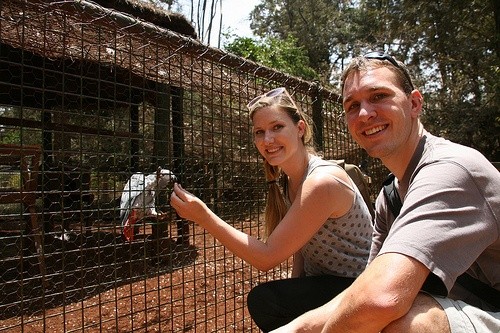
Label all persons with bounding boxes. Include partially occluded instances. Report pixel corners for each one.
[268,53,500,333]
[170,87,374,333]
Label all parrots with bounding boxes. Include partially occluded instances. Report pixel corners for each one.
[119,163,178,223]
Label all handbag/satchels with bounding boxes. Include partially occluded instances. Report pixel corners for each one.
[328,158,375,219]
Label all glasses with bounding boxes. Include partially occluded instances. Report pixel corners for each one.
[364,51,400,70]
[246,87,298,113]
